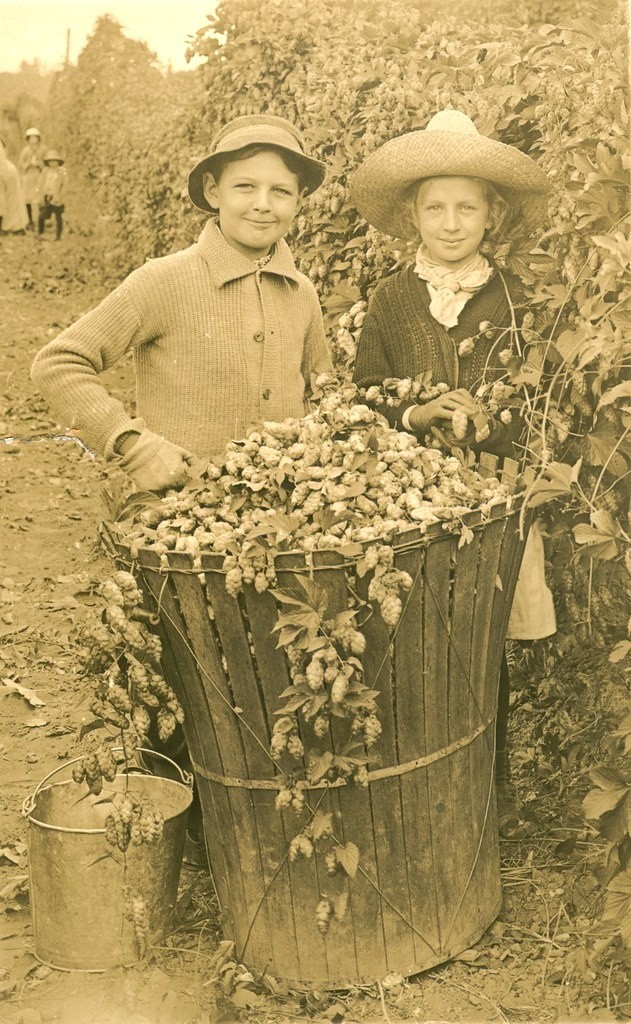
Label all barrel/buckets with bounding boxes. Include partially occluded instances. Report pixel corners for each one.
[21,746,194,971]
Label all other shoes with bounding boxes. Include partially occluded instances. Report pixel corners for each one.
[35,232,44,237]
[54,235,61,242]
[184,829,208,874]
[27,221,34,230]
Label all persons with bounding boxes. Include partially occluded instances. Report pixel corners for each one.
[345,107,560,840]
[30,114,337,868]
[0,126,65,240]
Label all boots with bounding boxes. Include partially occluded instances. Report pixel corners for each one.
[493,745,519,839]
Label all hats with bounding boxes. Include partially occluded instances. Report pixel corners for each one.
[22,128,42,139]
[352,111,552,248]
[44,150,66,167]
[187,115,327,214]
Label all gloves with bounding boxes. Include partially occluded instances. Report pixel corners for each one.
[407,388,478,434]
[429,412,508,456]
[114,426,198,494]
